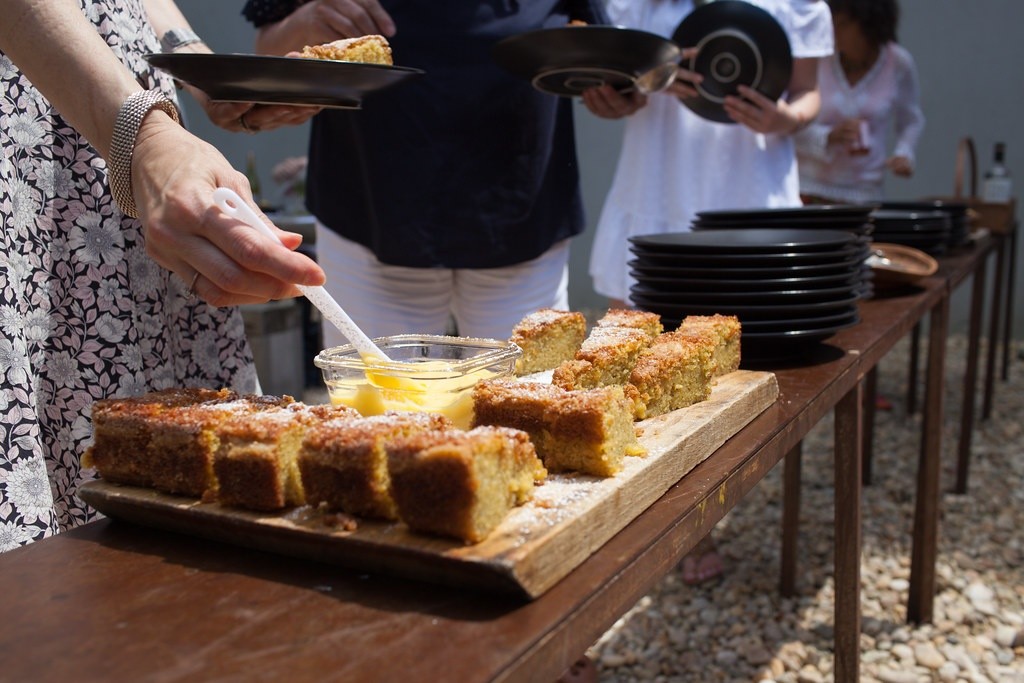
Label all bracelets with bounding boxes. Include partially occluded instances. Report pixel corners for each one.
[790,109,806,134]
[108,89,181,218]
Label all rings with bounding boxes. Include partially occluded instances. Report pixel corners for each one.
[181,271,199,299]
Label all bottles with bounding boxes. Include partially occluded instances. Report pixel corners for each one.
[980,142,1011,201]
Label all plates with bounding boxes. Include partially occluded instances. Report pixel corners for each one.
[479,26,681,98]
[627,200,972,348]
[141,52,424,109]
[671,0,793,124]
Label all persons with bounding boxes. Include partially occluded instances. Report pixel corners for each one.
[588,0,837,311]
[784,0,927,205]
[240,0,648,379]
[0,0,327,555]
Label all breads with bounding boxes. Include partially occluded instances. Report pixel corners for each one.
[79,306,742,546]
[298,34,394,65]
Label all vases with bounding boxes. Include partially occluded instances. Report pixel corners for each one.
[284,191,308,215]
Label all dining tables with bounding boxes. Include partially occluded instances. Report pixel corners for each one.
[2,204,1018,682]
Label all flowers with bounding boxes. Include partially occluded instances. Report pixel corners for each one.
[271,154,308,195]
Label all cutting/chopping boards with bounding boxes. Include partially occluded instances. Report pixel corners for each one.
[78,371,779,600]
